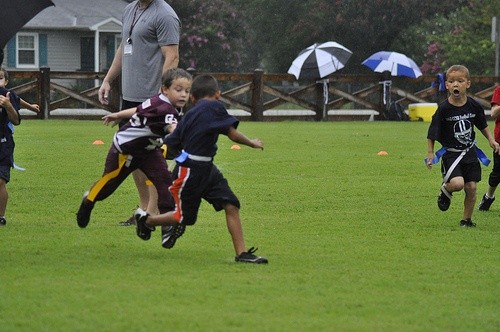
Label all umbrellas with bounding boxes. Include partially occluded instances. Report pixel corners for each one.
[0,0,57,57]
[361,49,424,79]
[287,40,354,80]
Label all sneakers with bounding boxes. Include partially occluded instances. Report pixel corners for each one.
[134,208,155,241]
[479,193,495,212]
[162,223,185,249]
[234,247,268,264]
[76,192,94,228]
[438,181,453,211]
[460,218,476,228]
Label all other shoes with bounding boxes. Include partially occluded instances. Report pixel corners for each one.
[119,216,135,226]
[0,217,6,224]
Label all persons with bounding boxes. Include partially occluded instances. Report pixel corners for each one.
[76,66,194,249]
[426,64,500,228]
[98,0,181,228]
[431,65,446,106]
[0,84,22,226]
[0,67,41,114]
[478,82,500,212]
[133,75,270,266]
